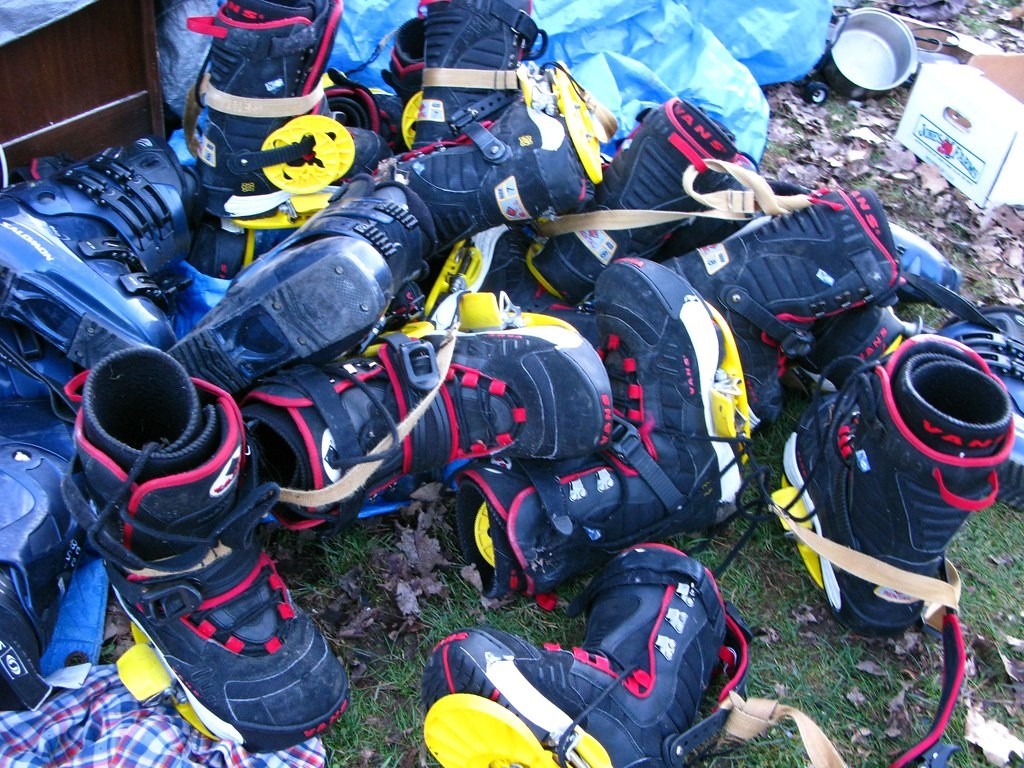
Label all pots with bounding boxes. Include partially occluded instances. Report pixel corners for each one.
[825,7,919,101]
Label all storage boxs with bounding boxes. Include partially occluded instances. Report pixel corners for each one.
[897,52,1024,208]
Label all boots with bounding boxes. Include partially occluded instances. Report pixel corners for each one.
[0,0,1024,768]
